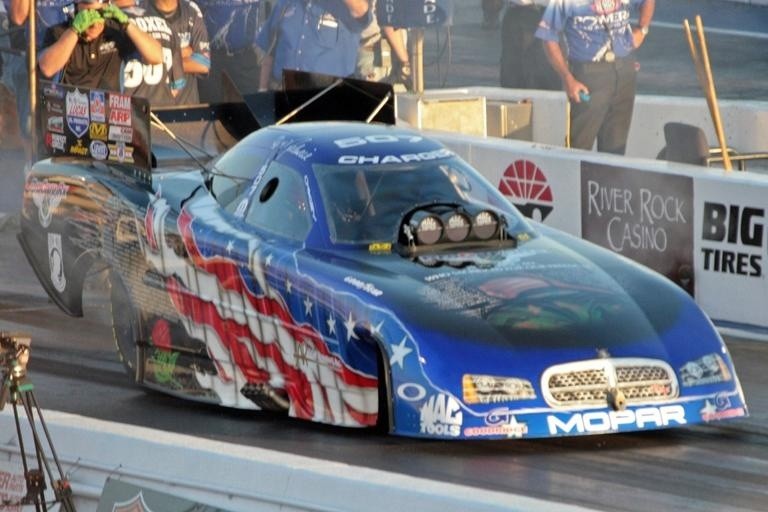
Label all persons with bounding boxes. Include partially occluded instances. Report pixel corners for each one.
[482,1,570,92]
[2,1,450,157]
[536,0,656,155]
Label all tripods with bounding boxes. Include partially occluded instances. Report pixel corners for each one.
[0,377,76,512]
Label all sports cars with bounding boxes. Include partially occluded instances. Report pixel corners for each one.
[13,67,754,443]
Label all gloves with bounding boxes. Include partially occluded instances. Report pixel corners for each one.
[72,3,128,35]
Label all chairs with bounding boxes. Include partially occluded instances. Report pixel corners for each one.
[654,123,743,173]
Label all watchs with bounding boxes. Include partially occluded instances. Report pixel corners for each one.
[638,25,651,34]
